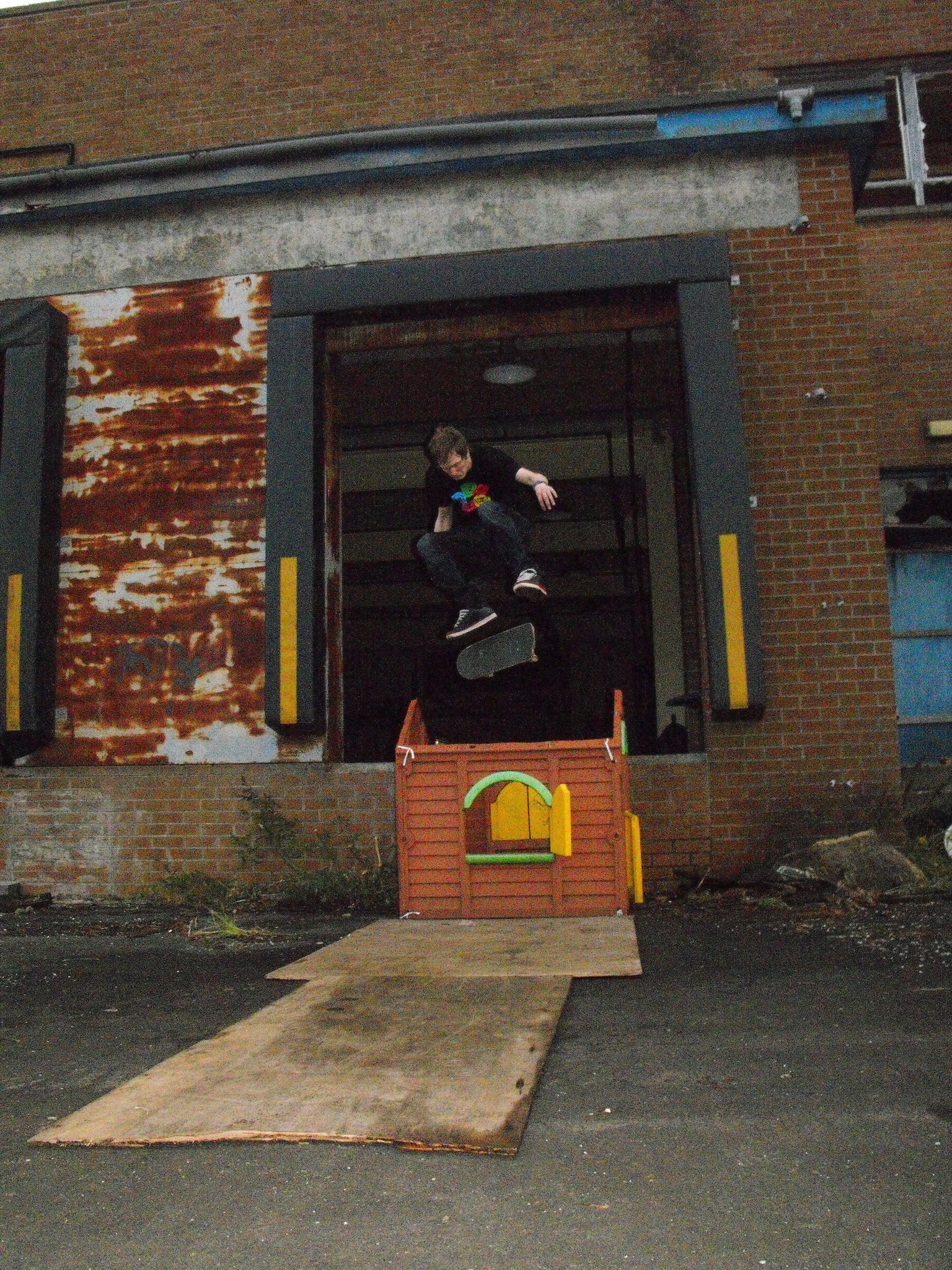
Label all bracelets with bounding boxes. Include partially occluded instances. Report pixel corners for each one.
[532,480,547,488]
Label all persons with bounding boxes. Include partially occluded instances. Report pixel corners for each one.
[415,425,558,639]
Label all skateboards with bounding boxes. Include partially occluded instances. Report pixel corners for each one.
[456,623,538,680]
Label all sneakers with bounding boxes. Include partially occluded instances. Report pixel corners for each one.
[446,607,497,639]
[513,569,547,602]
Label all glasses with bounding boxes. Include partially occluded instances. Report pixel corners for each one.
[442,459,464,473]
